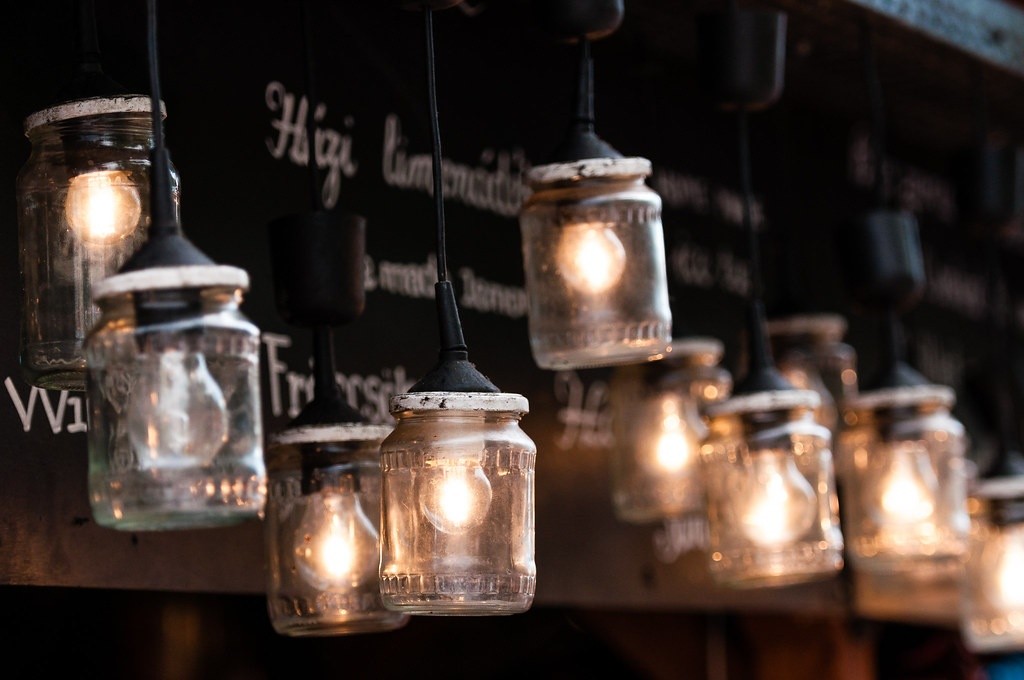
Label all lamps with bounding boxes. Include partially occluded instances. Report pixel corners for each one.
[14,0,1024,653]
